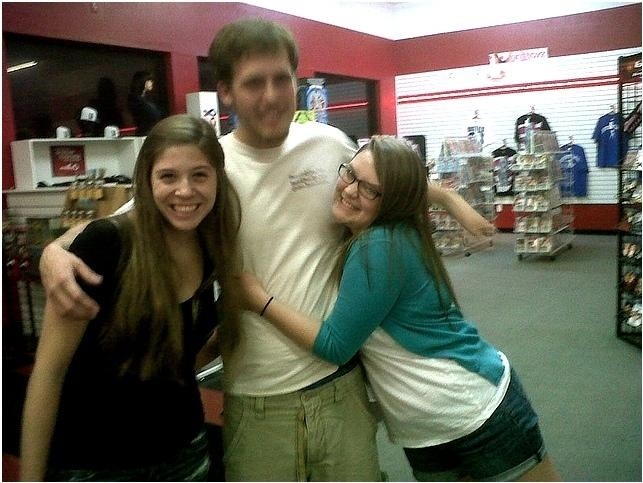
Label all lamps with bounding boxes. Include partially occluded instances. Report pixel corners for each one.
[7,60,39,74]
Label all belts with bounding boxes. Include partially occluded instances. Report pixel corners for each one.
[303,353,359,390]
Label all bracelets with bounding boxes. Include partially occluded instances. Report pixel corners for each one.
[259,296,273,316]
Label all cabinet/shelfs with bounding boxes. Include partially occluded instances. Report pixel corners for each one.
[2,135,147,339]
[430,137,497,258]
[614,55,642,342]
[509,130,575,261]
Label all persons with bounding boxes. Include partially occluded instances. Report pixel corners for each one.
[227,133,562,481]
[16,112,242,481]
[76,75,124,136]
[35,16,499,481]
[125,69,167,136]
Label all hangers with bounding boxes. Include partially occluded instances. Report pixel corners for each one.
[558,134,581,150]
[597,105,620,119]
[491,138,517,155]
[516,107,546,119]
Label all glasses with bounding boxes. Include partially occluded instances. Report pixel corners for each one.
[338,163,383,200]
